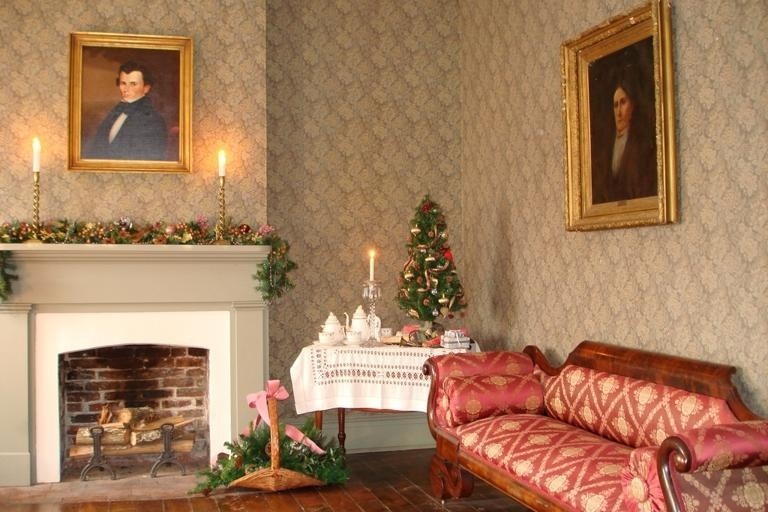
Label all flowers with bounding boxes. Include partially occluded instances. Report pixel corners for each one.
[0,213,299,308]
[187,419,353,497]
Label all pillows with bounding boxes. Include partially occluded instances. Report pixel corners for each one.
[438,370,548,430]
[620,445,670,511]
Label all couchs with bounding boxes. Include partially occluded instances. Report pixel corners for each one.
[421,338,768,512]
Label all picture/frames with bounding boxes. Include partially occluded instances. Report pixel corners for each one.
[557,0,682,233]
[66,28,197,176]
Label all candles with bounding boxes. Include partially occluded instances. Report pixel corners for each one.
[218,156,226,176]
[32,146,42,171]
[369,258,375,282]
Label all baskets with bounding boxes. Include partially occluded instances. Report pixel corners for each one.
[227,396,327,492]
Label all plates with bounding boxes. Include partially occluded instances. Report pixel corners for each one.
[313,340,338,346]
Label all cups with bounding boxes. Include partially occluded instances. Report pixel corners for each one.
[345,331,362,343]
[319,332,335,343]
[382,328,392,337]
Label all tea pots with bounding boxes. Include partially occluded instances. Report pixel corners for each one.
[343,305,371,343]
[320,312,345,343]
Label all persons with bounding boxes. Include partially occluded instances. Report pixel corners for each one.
[584,64,658,209]
[77,59,168,164]
